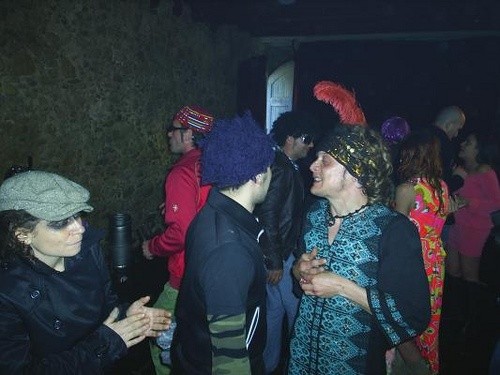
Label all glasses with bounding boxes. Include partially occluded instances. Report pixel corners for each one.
[29,212,83,231]
[168,124,184,133]
[294,135,315,146]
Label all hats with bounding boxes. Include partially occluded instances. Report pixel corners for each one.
[0,171,94,221]
[176,104,214,133]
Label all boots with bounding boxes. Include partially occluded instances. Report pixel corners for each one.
[441,275,463,327]
[448,279,481,328]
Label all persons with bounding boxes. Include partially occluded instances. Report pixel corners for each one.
[450,131,500,280]
[142,106,213,375]
[0,170,172,375]
[285,124,431,375]
[170,114,274,375]
[392,131,449,375]
[253,111,301,375]
[431,105,467,273]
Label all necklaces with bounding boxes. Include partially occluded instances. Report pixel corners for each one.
[327,203,368,226]
